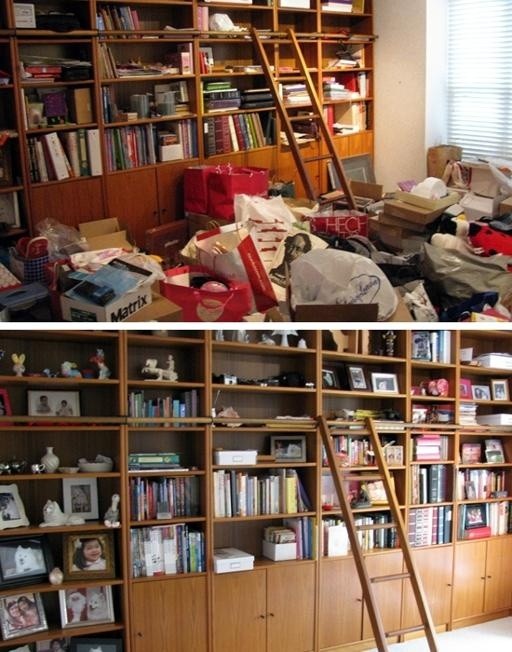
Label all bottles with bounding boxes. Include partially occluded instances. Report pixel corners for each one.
[0,454,27,475]
[297,338,307,349]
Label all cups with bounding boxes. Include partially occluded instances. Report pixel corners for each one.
[129,94,150,118]
[30,463,46,474]
[157,92,177,115]
[322,495,334,511]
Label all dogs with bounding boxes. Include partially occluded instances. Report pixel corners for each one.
[38,499,86,528]
[72,486,89,512]
[87,591,108,620]
[14,545,40,574]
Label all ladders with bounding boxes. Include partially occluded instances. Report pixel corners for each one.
[317,415,439,652]
[251,28,358,211]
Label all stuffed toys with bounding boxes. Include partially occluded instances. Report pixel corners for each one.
[11,347,111,380]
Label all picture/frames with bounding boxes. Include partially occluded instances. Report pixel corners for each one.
[23,386,86,420]
[0,535,57,590]
[60,477,101,522]
[70,635,124,651]
[1,585,49,643]
[63,533,117,580]
[0,387,17,428]
[58,582,117,629]
[0,482,30,530]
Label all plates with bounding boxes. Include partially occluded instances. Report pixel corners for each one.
[59,466,79,474]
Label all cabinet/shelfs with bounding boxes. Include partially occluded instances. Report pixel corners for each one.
[122,330,511,652]
[0,330,126,652]
[0,0,375,251]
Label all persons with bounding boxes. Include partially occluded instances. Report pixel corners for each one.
[71,537,106,572]
[55,399,73,416]
[36,395,50,413]
[49,639,65,652]
[7,595,41,632]
[268,233,312,288]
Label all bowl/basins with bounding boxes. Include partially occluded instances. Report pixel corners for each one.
[78,462,114,472]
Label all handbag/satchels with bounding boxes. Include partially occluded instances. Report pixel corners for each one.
[195,230,279,312]
[161,264,252,322]
[300,209,368,239]
[183,165,269,222]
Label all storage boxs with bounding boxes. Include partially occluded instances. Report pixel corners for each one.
[58,271,152,322]
[120,288,183,322]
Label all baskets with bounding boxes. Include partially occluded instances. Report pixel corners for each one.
[8,247,49,281]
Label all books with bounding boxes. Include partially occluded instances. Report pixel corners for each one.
[128,330,512,579]
[0,0,371,186]
